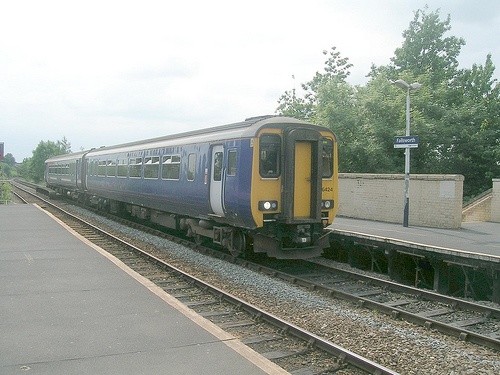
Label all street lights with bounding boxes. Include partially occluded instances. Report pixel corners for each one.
[396,78,420,228]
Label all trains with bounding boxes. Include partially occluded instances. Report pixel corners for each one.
[44,115,336,260]
